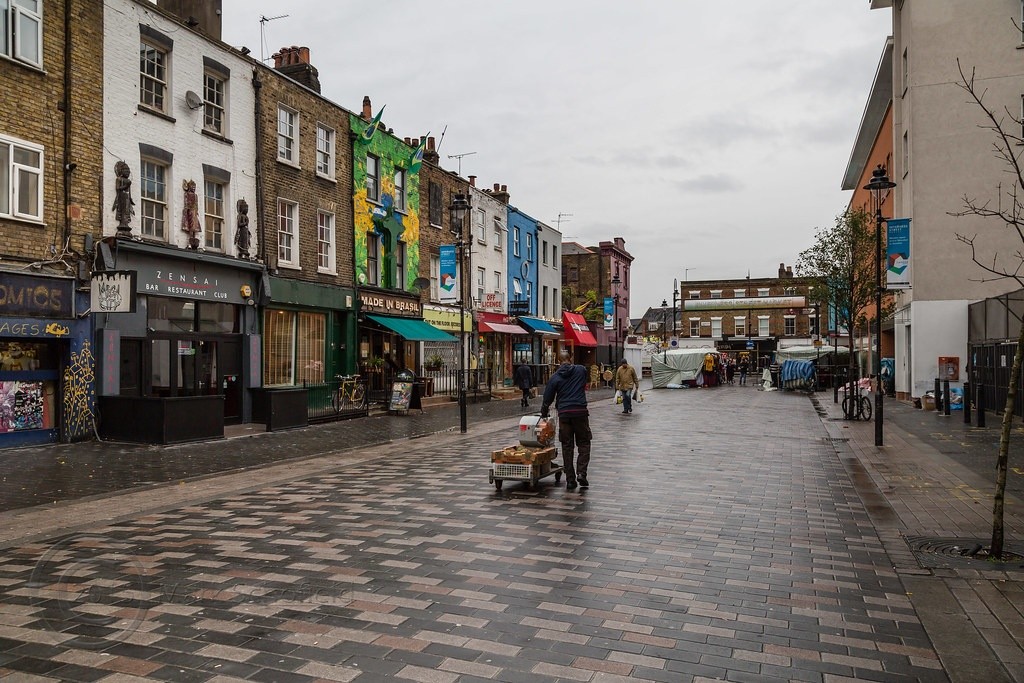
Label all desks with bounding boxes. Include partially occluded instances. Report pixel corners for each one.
[416,377,433,397]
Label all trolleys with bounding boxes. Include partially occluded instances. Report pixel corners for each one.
[489,445,564,489]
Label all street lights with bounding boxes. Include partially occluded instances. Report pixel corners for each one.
[448,189,473,434]
[862,164,897,447]
[610,272,624,368]
[673,279,679,336]
[660,299,669,364]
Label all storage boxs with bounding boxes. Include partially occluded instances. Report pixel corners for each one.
[491,450,547,466]
[922,397,936,410]
[504,444,558,460]
[493,463,540,479]
[541,461,552,473]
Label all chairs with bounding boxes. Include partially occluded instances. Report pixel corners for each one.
[589,364,601,390]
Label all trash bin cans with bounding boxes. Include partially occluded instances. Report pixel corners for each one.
[814,375,827,391]
[604,365,613,386]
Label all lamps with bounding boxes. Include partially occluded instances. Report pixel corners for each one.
[387,127,394,135]
[536,223,542,231]
[188,15,199,26]
[478,334,484,344]
[509,206,518,214]
[360,110,367,119]
[349,129,359,144]
[241,46,251,55]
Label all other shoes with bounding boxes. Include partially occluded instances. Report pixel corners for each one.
[629,408,632,411]
[525,402,529,407]
[521,399,525,407]
[622,411,628,413]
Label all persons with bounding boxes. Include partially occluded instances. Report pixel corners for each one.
[616,359,639,413]
[726,358,747,386]
[540,351,592,488]
[182,179,202,249]
[113,161,135,239]
[1,341,32,371]
[514,358,533,407]
[234,199,251,258]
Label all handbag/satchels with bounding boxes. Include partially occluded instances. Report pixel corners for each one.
[529,387,537,399]
[613,390,624,405]
[632,388,644,403]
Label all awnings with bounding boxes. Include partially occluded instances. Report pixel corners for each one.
[479,311,598,347]
[364,315,460,343]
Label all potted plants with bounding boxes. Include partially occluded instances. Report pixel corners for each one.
[422,355,444,371]
[361,354,385,373]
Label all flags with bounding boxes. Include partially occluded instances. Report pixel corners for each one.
[361,103,386,144]
[410,131,430,175]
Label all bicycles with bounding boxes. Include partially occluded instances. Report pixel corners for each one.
[842,385,872,421]
[332,374,366,412]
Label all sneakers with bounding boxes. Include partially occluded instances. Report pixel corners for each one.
[578,474,589,486]
[567,482,578,488]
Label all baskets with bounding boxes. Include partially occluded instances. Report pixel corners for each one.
[492,462,551,478]
